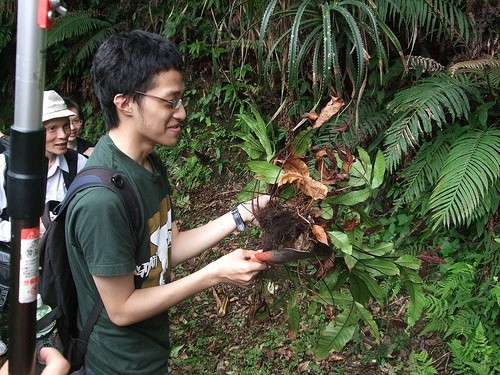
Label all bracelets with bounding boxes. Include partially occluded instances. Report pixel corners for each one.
[230,207,245,231]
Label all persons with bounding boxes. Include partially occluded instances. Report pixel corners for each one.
[0,90,93,375]
[64,30,279,375]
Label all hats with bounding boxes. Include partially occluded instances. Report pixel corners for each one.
[42,90,76,123]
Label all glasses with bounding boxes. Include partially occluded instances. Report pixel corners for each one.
[69,120,83,126]
[132,90,190,109]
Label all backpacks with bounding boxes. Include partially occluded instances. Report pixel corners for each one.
[39,168,140,373]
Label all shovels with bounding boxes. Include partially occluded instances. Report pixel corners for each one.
[246,247,310,264]
